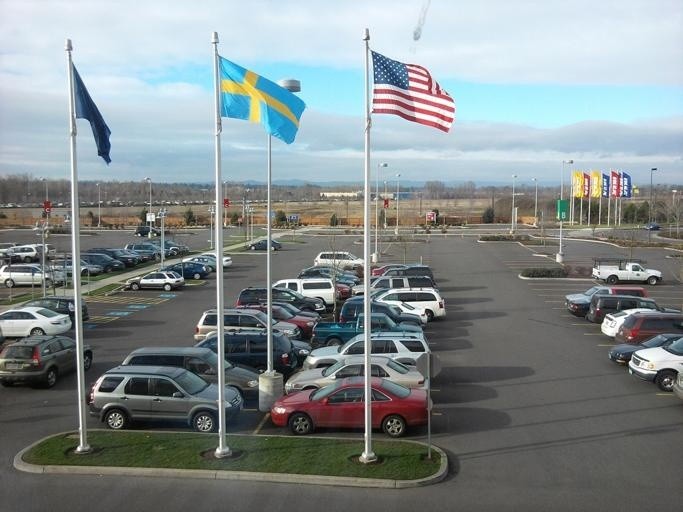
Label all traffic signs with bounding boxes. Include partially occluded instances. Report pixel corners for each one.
[287,214,299,224]
[426,211,436,222]
[266,76,301,373]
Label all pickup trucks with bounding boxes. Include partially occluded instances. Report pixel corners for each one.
[313,313,424,346]
[592,261,662,286]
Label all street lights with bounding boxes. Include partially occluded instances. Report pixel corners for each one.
[556,159,574,262]
[242,187,250,224]
[207,205,216,249]
[532,177,538,225]
[648,168,658,243]
[157,211,166,269]
[649,167,657,227]
[225,179,228,227]
[208,206,215,250]
[375,161,388,254]
[223,179,228,228]
[143,175,153,240]
[532,177,538,226]
[35,219,47,297]
[249,207,255,242]
[372,160,389,263]
[40,177,48,226]
[395,171,400,234]
[96,183,100,225]
[156,208,169,265]
[248,207,256,244]
[33,221,51,299]
[559,159,573,253]
[95,183,102,229]
[144,177,152,232]
[39,176,48,237]
[159,208,169,259]
[258,78,302,413]
[395,173,400,234]
[384,180,388,224]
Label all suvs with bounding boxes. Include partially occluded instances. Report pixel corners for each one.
[86,365,243,432]
[56,260,103,277]
[565,287,647,316]
[628,337,683,391]
[587,294,681,325]
[136,226,162,237]
[122,347,265,401]
[615,311,683,344]
[236,287,326,314]
[194,332,298,375]
[88,248,138,267]
[376,287,446,322]
[303,331,431,378]
[314,251,364,269]
[192,309,302,341]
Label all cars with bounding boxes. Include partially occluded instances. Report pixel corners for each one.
[601,308,653,337]
[335,283,352,299]
[371,263,408,275]
[0,306,73,338]
[160,262,212,280]
[291,339,312,369]
[305,268,360,284]
[608,333,683,366]
[246,239,281,251]
[390,302,428,324]
[297,264,359,279]
[126,271,185,291]
[305,273,354,287]
[182,253,232,267]
[71,252,125,273]
[645,222,660,231]
[121,248,142,264]
[285,356,428,396]
[126,249,150,261]
[271,375,434,437]
[270,301,322,321]
[188,256,216,272]
[0,250,22,266]
[144,239,189,255]
[236,304,317,335]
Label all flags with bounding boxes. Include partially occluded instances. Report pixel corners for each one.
[218,53,307,145]
[72,62,112,166]
[370,48,456,134]
[571,170,630,198]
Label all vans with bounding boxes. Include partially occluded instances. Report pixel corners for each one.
[0,335,93,388]
[126,244,172,261]
[31,243,55,257]
[8,245,42,263]
[0,263,66,289]
[339,300,422,326]
[272,279,334,311]
[370,266,434,284]
[351,276,435,294]
[18,297,89,324]
